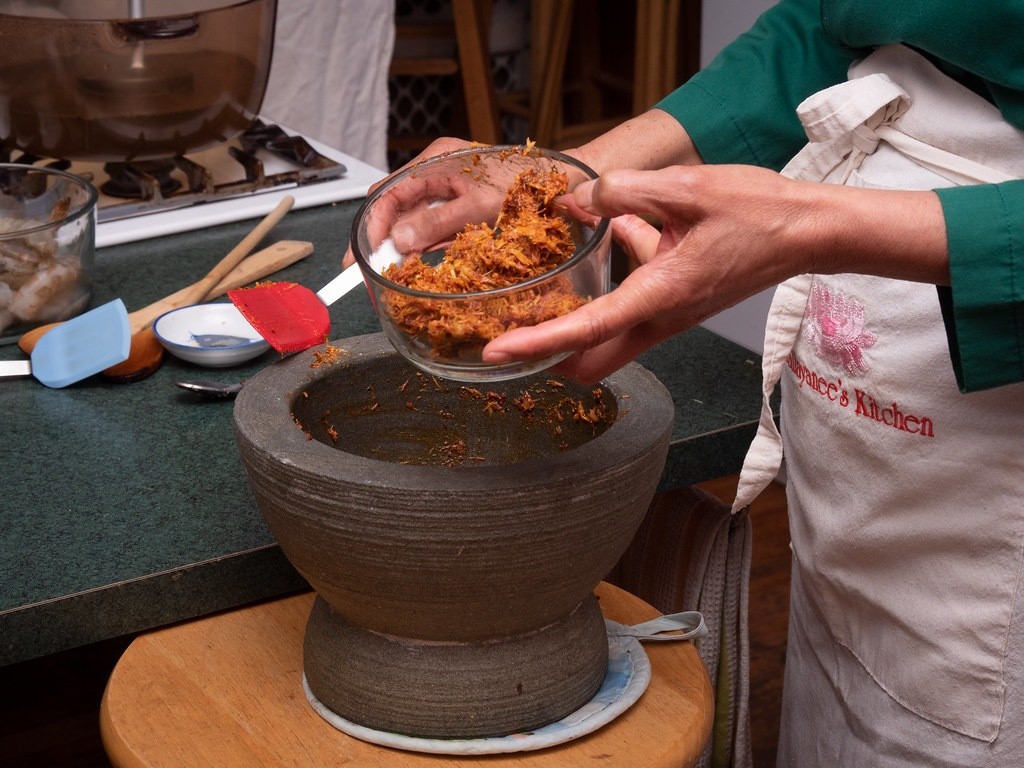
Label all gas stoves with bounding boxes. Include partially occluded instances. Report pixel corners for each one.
[0,119,348,223]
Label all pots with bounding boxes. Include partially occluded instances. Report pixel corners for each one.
[0,0,279,163]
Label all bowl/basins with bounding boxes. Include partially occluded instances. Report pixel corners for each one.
[349,145,613,384]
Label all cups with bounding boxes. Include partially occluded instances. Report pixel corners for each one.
[0,162,101,346]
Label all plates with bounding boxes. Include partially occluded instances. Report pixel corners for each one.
[153,300,272,368]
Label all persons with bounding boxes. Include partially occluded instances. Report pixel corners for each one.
[340,0,1024,768]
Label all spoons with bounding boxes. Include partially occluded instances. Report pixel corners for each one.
[104,195,294,384]
[173,377,243,398]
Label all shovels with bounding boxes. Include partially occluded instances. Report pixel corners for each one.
[17,239,314,354]
[0,297,130,387]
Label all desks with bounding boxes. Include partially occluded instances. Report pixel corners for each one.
[90,563,719,768]
